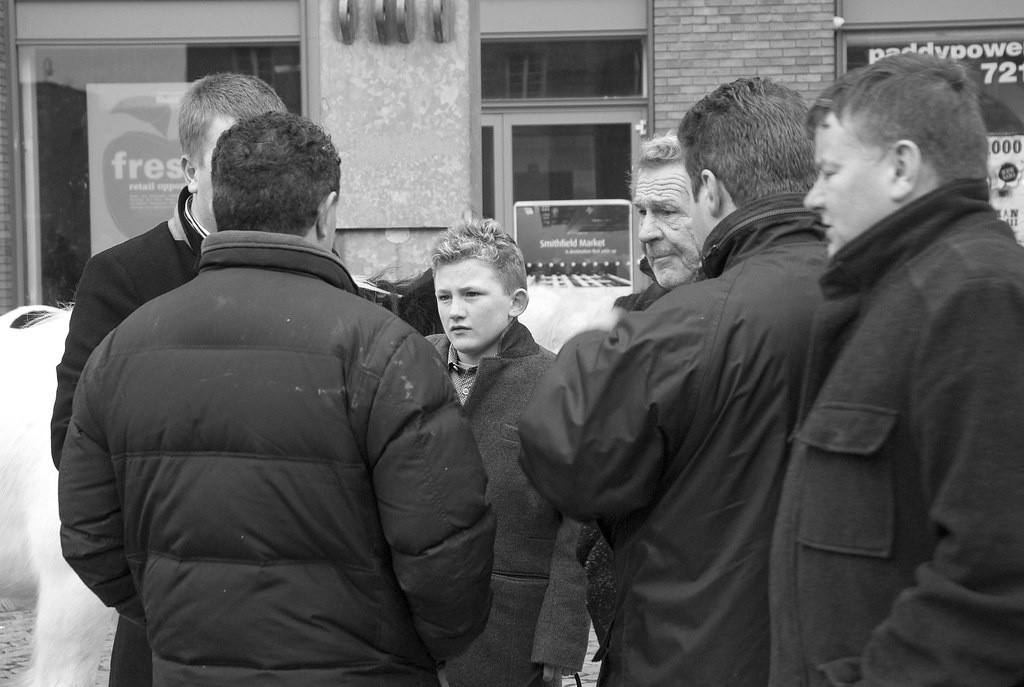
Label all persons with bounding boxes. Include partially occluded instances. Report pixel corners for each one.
[59,111,496,687]
[51,73,340,687]
[419,216,591,687]
[576,134,701,643]
[519,76,830,687]
[770,52,1024,687]
